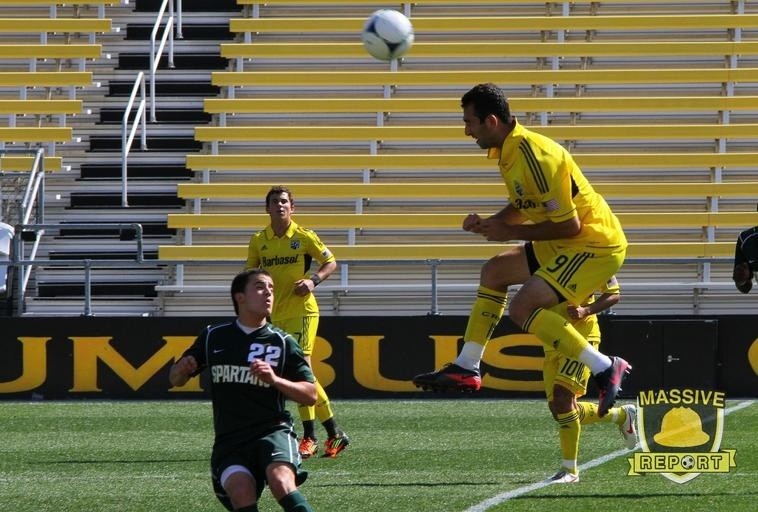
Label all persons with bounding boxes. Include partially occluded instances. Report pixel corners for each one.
[168,268,319,512]
[542,274,636,485]
[412,83,633,419]
[243,186,350,461]
[0,221,16,316]
[731,202,758,294]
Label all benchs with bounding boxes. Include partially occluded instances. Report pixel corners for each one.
[0,4,758,283]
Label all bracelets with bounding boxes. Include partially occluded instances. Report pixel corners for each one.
[309,273,322,287]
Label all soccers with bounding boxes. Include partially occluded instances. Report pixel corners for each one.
[362,10,414,60]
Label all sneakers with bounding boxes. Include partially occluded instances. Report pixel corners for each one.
[413,363,481,392]
[299,437,318,458]
[545,471,579,483]
[320,432,349,457]
[595,356,637,450]
